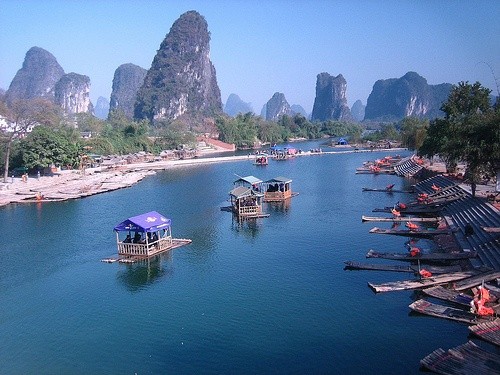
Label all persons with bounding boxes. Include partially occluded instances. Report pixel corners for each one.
[236,199,258,213]
[266,184,287,192]
[252,184,258,191]
[122,232,158,253]
[256,156,265,163]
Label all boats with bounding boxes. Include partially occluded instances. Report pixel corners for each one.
[368,248,477,260]
[385,202,445,208]
[362,216,442,221]
[405,192,453,202]
[360,184,417,194]
[99,210,192,264]
[218,186,275,219]
[230,174,261,197]
[344,260,464,273]
[409,193,464,204]
[258,177,298,202]
[418,275,500,375]
[252,151,270,166]
[269,144,297,161]
[367,224,453,236]
[370,273,469,293]
[417,181,456,196]
[354,155,398,174]
[372,207,445,214]
